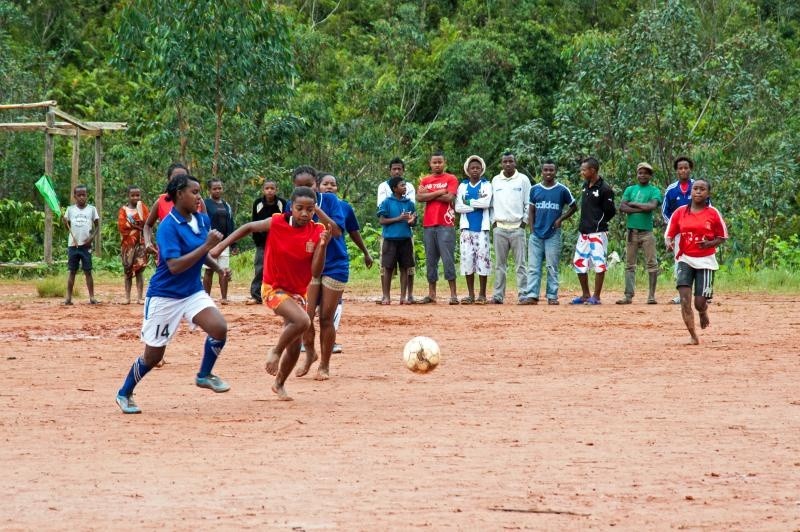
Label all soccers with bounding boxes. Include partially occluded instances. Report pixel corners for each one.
[402,335,441,374]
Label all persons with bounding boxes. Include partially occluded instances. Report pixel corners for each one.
[378,154,711,306]
[663,178,729,345]
[62,162,373,418]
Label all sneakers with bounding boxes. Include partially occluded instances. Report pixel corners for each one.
[116,392,141,414]
[196,373,231,392]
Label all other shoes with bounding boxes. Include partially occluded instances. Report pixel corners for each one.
[475,295,488,304]
[672,296,682,304]
[300,343,306,351]
[615,297,632,304]
[487,297,502,304]
[518,295,527,304]
[548,298,559,305]
[647,298,657,304]
[524,297,538,305]
[333,342,342,353]
[461,297,475,305]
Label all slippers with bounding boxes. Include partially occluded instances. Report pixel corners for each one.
[449,297,459,305]
[420,297,436,303]
[569,297,584,304]
[584,297,602,305]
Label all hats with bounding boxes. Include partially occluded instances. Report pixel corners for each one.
[637,162,652,170]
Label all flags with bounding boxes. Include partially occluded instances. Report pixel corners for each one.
[35,174,61,220]
